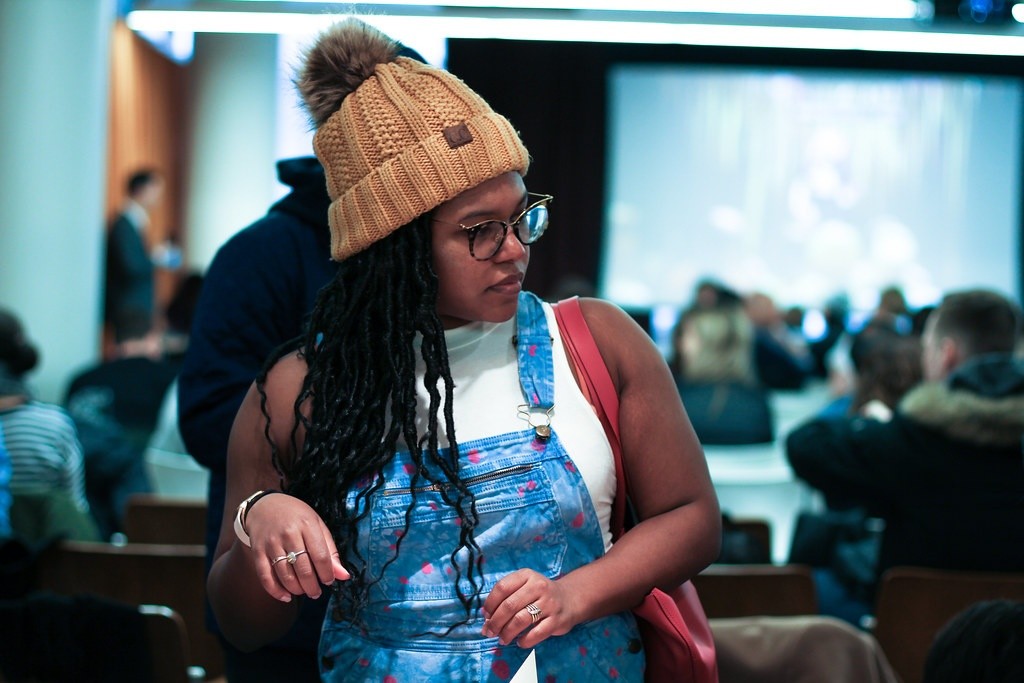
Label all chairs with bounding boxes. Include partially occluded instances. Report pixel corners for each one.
[0,494,1024,683]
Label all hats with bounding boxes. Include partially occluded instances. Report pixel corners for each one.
[302,21,532,263]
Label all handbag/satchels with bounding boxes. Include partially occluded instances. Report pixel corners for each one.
[633,578,718,683]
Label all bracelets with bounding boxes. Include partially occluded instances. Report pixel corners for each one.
[233,490,284,547]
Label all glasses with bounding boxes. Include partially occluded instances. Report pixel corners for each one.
[432,191,553,261]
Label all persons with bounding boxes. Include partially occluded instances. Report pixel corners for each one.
[105,166,183,355]
[59,267,203,543]
[178,40,429,682]
[205,28,722,683]
[673,284,935,447]
[784,290,1024,624]
[1,307,98,541]
[924,600,1024,683]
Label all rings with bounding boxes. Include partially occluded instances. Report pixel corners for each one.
[525,604,542,623]
[272,555,287,566]
[286,550,308,564]
